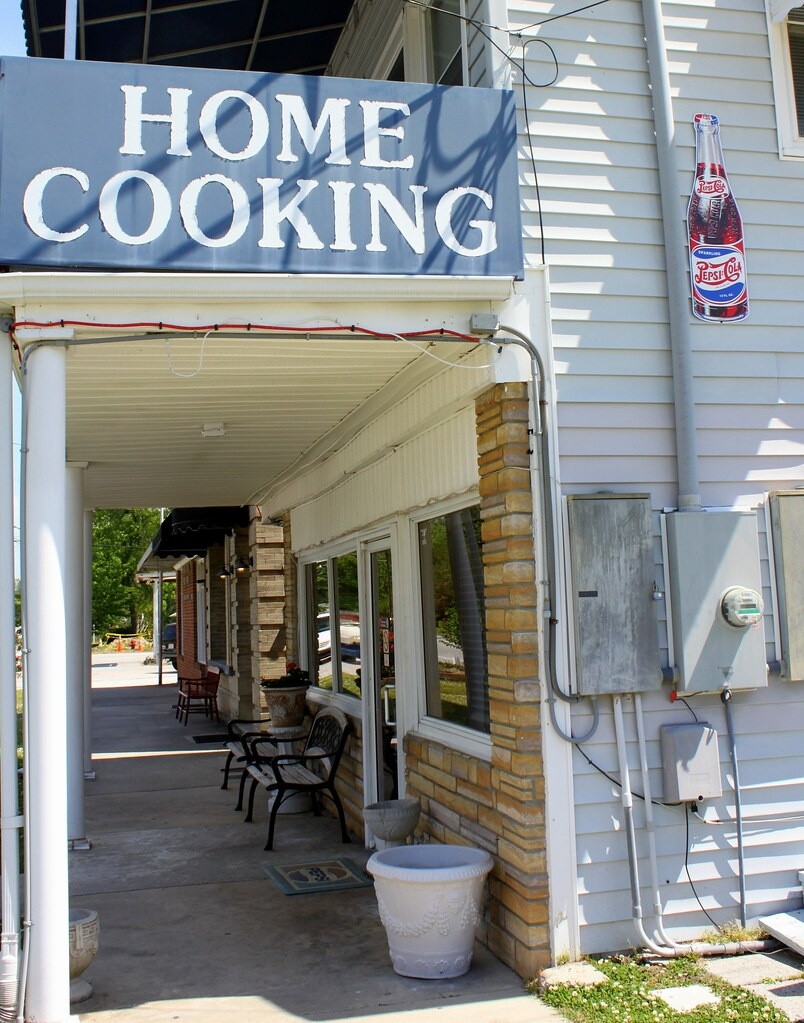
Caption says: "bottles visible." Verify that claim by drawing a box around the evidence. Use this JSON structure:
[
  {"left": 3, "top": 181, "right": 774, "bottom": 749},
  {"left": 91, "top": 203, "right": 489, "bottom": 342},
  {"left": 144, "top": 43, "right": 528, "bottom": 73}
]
[{"left": 687, "top": 114, "right": 750, "bottom": 323}]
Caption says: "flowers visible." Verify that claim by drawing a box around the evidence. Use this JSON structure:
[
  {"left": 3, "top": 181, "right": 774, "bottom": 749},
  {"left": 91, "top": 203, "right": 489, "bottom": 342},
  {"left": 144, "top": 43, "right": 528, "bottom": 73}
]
[{"left": 260, "top": 662, "right": 313, "bottom": 687}]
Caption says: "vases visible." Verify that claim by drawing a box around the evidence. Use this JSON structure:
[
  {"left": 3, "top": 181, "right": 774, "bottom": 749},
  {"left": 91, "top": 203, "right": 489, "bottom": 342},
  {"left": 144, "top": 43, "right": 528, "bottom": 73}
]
[
  {"left": 262, "top": 686, "right": 309, "bottom": 726},
  {"left": 366, "top": 842, "right": 495, "bottom": 979},
  {"left": 362, "top": 797, "right": 422, "bottom": 851},
  {"left": 68, "top": 910, "right": 100, "bottom": 1002}
]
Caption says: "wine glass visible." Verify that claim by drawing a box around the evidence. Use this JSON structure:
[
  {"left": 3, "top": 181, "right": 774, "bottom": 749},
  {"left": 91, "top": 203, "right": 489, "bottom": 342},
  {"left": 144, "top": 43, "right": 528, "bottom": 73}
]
[
  {"left": 363, "top": 799, "right": 421, "bottom": 849},
  {"left": 70, "top": 909, "right": 99, "bottom": 1002}
]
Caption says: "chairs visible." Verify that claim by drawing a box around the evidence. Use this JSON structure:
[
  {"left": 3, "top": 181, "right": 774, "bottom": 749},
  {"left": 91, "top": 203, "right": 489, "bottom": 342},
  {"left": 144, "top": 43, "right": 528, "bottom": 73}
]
[
  {"left": 221, "top": 718, "right": 275, "bottom": 810},
  {"left": 175, "top": 667, "right": 223, "bottom": 725},
  {"left": 235, "top": 708, "right": 352, "bottom": 852}
]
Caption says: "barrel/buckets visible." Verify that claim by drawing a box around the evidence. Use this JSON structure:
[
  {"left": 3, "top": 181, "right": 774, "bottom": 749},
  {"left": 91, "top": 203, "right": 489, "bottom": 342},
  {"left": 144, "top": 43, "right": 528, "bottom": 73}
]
[{"left": 366, "top": 845, "right": 495, "bottom": 979}]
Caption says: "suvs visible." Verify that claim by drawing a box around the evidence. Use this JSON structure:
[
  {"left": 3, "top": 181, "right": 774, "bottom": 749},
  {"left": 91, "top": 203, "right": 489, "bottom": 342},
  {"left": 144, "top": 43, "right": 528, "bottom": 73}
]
[
  {"left": 161, "top": 623, "right": 177, "bottom": 669},
  {"left": 316, "top": 612, "right": 331, "bottom": 659},
  {"left": 338, "top": 610, "right": 360, "bottom": 656}
]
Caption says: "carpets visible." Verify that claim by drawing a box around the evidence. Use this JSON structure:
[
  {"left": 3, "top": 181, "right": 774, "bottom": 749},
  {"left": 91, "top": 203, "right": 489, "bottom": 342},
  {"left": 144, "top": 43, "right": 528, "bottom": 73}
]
[
  {"left": 264, "top": 855, "right": 376, "bottom": 897},
  {"left": 192, "top": 732, "right": 239, "bottom": 744}
]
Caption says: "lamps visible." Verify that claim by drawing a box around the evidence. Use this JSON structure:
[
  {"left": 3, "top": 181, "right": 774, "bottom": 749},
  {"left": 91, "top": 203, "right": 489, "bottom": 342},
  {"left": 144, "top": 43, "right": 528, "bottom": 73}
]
[
  {"left": 236, "top": 556, "right": 254, "bottom": 573},
  {"left": 220, "top": 563, "right": 232, "bottom": 578}
]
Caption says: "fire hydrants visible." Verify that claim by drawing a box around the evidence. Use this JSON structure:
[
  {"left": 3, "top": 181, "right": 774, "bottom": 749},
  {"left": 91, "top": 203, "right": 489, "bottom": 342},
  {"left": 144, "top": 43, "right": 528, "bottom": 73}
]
[{"left": 130, "top": 639, "right": 136, "bottom": 649}]
